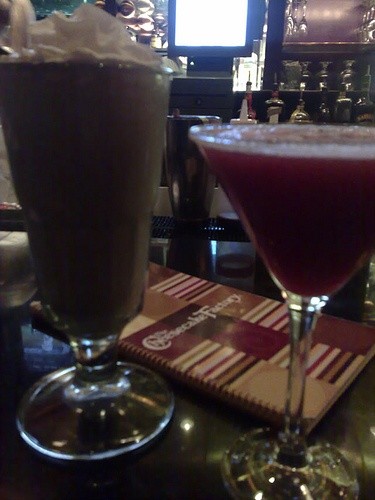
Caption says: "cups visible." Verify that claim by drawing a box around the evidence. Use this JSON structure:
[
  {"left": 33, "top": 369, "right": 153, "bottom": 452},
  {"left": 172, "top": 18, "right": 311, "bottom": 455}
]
[{"left": 166, "top": 115, "right": 222, "bottom": 227}]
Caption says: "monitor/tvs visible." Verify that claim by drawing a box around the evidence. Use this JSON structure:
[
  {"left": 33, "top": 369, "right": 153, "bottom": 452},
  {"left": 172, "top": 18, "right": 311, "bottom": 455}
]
[{"left": 167, "top": 0, "right": 255, "bottom": 73}]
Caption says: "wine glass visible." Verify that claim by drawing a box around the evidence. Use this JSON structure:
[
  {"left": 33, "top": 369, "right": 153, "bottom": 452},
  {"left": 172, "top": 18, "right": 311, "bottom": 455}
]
[
  {"left": 0, "top": 63, "right": 173, "bottom": 460},
  {"left": 190, "top": 124, "right": 375, "bottom": 500}
]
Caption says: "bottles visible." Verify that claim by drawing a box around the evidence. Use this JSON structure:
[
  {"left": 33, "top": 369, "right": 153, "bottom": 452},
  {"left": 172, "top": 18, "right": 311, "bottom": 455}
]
[
  {"left": 334, "top": 83, "right": 350, "bottom": 122},
  {"left": 150, "top": 30, "right": 157, "bottom": 48},
  {"left": 318, "top": 86, "right": 329, "bottom": 122},
  {"left": 265, "top": 82, "right": 283, "bottom": 121},
  {"left": 290, "top": 99, "right": 310, "bottom": 121}
]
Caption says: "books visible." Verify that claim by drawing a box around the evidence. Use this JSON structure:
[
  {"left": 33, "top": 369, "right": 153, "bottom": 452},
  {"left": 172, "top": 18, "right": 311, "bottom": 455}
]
[{"left": 27, "top": 256, "right": 375, "bottom": 435}]
